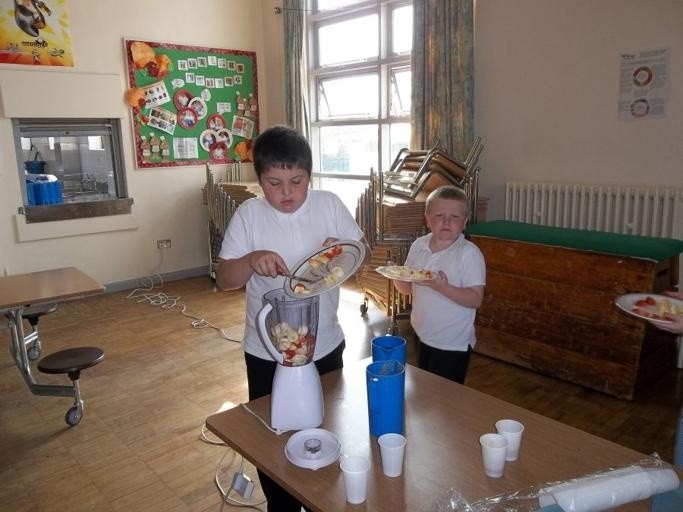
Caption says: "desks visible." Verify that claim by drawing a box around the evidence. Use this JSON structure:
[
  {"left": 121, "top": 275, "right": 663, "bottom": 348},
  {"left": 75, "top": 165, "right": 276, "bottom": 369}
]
[
  {"left": 206, "top": 351, "right": 683, "bottom": 512},
  {"left": 0, "top": 267, "right": 106, "bottom": 426}
]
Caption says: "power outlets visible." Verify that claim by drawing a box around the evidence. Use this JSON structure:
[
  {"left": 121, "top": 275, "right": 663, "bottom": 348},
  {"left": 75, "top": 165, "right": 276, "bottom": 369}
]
[{"left": 157, "top": 239, "right": 171, "bottom": 249}]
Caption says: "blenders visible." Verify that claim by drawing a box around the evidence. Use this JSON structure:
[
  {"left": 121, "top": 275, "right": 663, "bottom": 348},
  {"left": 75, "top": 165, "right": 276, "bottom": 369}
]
[{"left": 254, "top": 287, "right": 325, "bottom": 435}]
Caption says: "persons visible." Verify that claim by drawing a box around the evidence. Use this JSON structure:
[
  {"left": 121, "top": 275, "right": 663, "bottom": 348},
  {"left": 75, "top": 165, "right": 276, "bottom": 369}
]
[
  {"left": 214, "top": 126, "right": 373, "bottom": 512},
  {"left": 650, "top": 289, "right": 683, "bottom": 420},
  {"left": 387, "top": 187, "right": 487, "bottom": 385}
]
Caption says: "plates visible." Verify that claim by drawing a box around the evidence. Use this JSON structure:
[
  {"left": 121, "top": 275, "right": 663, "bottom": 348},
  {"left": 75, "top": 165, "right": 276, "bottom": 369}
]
[
  {"left": 374, "top": 265, "right": 440, "bottom": 283},
  {"left": 282, "top": 238, "right": 366, "bottom": 300},
  {"left": 612, "top": 292, "right": 682, "bottom": 324}
]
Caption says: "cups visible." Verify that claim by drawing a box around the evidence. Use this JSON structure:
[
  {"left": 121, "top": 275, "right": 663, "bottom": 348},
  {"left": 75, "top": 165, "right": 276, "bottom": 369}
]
[
  {"left": 477, "top": 419, "right": 525, "bottom": 480},
  {"left": 536, "top": 466, "right": 680, "bottom": 512},
  {"left": 339, "top": 454, "right": 369, "bottom": 505},
  {"left": 376, "top": 432, "right": 407, "bottom": 479}
]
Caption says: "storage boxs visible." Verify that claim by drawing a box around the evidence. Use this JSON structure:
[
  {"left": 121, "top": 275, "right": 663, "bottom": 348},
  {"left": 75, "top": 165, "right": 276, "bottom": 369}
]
[{"left": 26, "top": 180, "right": 63, "bottom": 207}]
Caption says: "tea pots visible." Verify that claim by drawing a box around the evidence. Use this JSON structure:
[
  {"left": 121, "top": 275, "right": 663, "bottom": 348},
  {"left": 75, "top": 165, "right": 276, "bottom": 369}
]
[{"left": 364, "top": 333, "right": 407, "bottom": 438}]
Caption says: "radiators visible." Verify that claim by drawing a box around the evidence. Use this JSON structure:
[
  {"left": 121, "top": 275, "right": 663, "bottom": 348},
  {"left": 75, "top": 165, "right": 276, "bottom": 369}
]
[{"left": 504, "top": 182, "right": 680, "bottom": 239}]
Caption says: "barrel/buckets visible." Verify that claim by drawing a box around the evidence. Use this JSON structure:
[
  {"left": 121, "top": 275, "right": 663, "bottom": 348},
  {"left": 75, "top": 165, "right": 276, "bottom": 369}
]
[{"left": 24, "top": 161, "right": 46, "bottom": 174}]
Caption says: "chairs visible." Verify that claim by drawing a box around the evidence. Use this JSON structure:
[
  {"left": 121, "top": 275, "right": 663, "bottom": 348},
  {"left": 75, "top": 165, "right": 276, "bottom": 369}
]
[{"left": 383, "top": 136, "right": 484, "bottom": 199}]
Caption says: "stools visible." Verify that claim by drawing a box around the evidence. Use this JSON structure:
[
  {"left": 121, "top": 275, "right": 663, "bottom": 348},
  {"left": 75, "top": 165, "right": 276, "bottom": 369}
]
[
  {"left": 3, "top": 302, "right": 58, "bottom": 361},
  {"left": 38, "top": 347, "right": 105, "bottom": 427}
]
